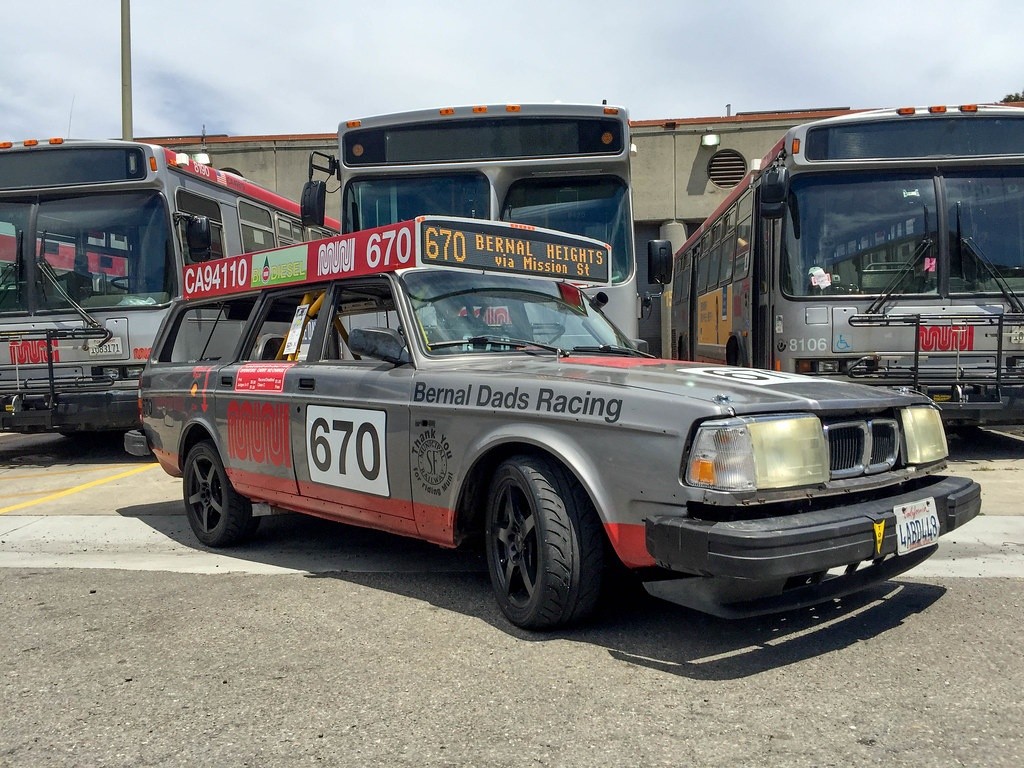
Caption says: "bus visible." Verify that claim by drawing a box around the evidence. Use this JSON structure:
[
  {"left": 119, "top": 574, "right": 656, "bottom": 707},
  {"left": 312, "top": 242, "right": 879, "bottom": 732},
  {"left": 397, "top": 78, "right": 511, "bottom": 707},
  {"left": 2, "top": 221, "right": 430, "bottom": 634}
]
[
  {"left": 0, "top": 212, "right": 130, "bottom": 295},
  {"left": 669, "top": 98, "right": 1024, "bottom": 429},
  {"left": 1, "top": 135, "right": 343, "bottom": 441},
  {"left": 299, "top": 102, "right": 676, "bottom": 352}
]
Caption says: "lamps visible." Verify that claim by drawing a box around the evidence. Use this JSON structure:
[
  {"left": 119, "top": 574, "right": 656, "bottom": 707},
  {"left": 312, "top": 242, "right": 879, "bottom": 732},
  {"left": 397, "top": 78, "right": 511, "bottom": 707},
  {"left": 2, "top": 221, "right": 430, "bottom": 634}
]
[
  {"left": 191, "top": 152, "right": 211, "bottom": 167},
  {"left": 700, "top": 133, "right": 720, "bottom": 146}
]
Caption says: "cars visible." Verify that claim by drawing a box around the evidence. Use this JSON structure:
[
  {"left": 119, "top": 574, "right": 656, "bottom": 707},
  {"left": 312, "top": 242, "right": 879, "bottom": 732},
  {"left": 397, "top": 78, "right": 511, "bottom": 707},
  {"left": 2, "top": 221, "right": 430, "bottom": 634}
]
[{"left": 121, "top": 214, "right": 984, "bottom": 638}]
